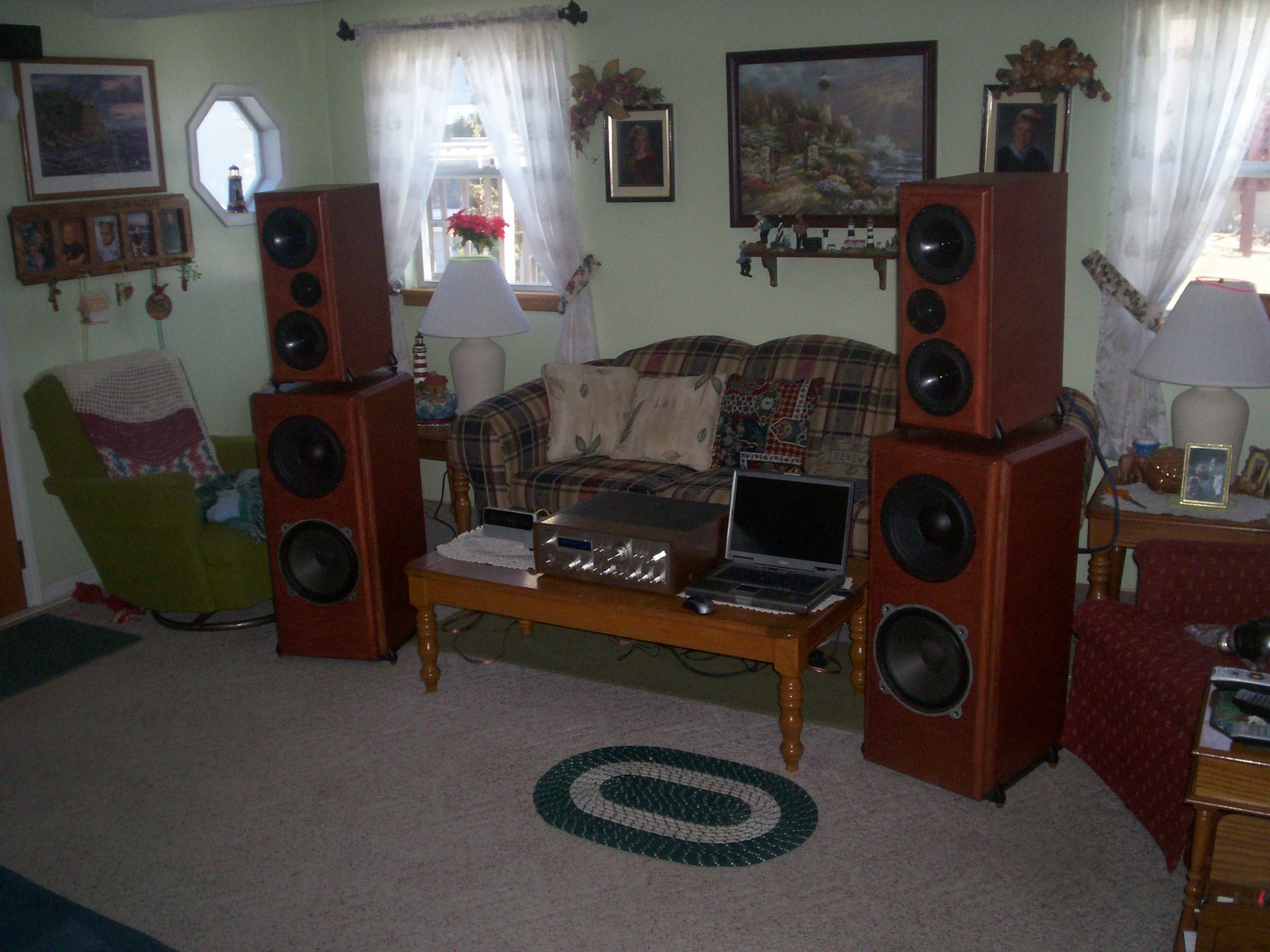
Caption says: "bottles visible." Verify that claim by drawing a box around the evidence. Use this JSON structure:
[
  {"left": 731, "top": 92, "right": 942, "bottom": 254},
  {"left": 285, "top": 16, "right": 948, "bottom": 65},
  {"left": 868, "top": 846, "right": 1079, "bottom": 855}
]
[
  {"left": 227, "top": 165, "right": 248, "bottom": 214},
  {"left": 413, "top": 331, "right": 428, "bottom": 383}
]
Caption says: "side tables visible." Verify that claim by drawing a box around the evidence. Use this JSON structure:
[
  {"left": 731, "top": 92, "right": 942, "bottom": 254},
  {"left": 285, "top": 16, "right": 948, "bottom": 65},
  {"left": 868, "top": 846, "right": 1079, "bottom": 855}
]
[{"left": 418, "top": 420, "right": 474, "bottom": 534}]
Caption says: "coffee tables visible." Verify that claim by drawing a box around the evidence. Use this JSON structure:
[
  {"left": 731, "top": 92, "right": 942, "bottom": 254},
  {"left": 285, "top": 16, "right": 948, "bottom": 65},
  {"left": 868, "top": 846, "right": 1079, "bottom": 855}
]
[{"left": 404, "top": 519, "right": 868, "bottom": 774}]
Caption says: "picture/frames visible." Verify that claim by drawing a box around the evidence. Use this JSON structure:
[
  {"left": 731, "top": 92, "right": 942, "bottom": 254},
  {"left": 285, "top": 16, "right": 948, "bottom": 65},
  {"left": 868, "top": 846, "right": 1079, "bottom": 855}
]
[
  {"left": 9, "top": 57, "right": 168, "bottom": 200},
  {"left": 1237, "top": 445, "right": 1270, "bottom": 497},
  {"left": 1178, "top": 442, "right": 1232, "bottom": 508},
  {"left": 725, "top": 40, "right": 937, "bottom": 228},
  {"left": 3, "top": 193, "right": 196, "bottom": 288},
  {"left": 605, "top": 101, "right": 674, "bottom": 204},
  {"left": 977, "top": 83, "right": 1075, "bottom": 173}
]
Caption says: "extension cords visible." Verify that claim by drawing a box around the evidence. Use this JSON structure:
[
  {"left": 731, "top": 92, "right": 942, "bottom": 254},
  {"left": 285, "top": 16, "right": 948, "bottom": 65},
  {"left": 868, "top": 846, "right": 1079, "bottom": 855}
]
[{"left": 481, "top": 507, "right": 536, "bottom": 533}]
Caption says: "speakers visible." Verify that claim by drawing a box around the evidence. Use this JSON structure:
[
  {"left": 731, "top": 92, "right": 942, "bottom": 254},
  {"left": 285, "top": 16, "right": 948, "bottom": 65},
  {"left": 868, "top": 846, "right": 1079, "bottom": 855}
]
[
  {"left": 252, "top": 181, "right": 400, "bottom": 391},
  {"left": 854, "top": 424, "right": 1093, "bottom": 809},
  {"left": 894, "top": 167, "right": 1068, "bottom": 439},
  {"left": 247, "top": 370, "right": 428, "bottom": 665}
]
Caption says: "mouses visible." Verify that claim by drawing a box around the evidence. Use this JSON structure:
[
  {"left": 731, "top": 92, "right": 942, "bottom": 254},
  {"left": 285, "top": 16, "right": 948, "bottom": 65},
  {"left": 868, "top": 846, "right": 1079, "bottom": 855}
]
[{"left": 683, "top": 594, "right": 716, "bottom": 615}]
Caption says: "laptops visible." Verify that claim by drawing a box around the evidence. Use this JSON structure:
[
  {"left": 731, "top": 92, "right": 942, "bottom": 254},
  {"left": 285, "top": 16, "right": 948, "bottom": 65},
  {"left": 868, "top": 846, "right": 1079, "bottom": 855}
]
[{"left": 683, "top": 469, "right": 856, "bottom": 616}]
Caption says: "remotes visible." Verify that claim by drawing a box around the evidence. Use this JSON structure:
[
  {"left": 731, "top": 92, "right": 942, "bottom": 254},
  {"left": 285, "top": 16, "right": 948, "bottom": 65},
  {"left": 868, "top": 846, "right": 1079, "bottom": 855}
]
[
  {"left": 1230, "top": 721, "right": 1270, "bottom": 744},
  {"left": 1232, "top": 690, "right": 1270, "bottom": 711},
  {"left": 1209, "top": 665, "right": 1270, "bottom": 693}
]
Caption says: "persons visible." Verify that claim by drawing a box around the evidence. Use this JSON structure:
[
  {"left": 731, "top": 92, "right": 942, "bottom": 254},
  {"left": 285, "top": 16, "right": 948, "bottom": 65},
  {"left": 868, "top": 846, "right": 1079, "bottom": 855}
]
[
  {"left": 751, "top": 211, "right": 772, "bottom": 243},
  {"left": 59, "top": 221, "right": 87, "bottom": 267},
  {"left": 22, "top": 224, "right": 53, "bottom": 270},
  {"left": 794, "top": 213, "right": 809, "bottom": 249},
  {"left": 97, "top": 221, "right": 120, "bottom": 261},
  {"left": 163, "top": 210, "right": 179, "bottom": 253},
  {"left": 736, "top": 241, "right": 752, "bottom": 278},
  {"left": 131, "top": 225, "right": 145, "bottom": 257},
  {"left": 618, "top": 124, "right": 663, "bottom": 186},
  {"left": 1186, "top": 457, "right": 1223, "bottom": 503}
]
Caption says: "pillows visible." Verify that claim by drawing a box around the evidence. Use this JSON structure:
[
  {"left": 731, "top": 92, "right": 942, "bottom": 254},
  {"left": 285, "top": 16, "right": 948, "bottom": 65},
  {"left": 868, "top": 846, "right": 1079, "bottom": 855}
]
[
  {"left": 537, "top": 358, "right": 639, "bottom": 462},
  {"left": 611, "top": 372, "right": 727, "bottom": 473},
  {"left": 705, "top": 374, "right": 827, "bottom": 478}
]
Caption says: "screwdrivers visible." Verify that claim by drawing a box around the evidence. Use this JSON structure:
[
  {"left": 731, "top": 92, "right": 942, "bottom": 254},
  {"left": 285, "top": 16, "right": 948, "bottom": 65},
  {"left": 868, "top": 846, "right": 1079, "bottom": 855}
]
[{"left": 1105, "top": 485, "right": 1147, "bottom": 509}]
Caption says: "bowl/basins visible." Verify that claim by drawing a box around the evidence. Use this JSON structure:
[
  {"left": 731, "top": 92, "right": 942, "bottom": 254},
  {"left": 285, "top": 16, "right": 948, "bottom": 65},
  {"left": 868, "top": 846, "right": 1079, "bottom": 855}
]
[{"left": 415, "top": 391, "right": 459, "bottom": 420}]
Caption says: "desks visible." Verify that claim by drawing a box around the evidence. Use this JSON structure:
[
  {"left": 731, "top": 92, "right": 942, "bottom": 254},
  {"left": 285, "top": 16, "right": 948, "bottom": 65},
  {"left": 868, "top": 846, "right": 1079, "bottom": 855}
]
[
  {"left": 1169, "top": 663, "right": 1270, "bottom": 952},
  {"left": 1084, "top": 462, "right": 1270, "bottom": 602}
]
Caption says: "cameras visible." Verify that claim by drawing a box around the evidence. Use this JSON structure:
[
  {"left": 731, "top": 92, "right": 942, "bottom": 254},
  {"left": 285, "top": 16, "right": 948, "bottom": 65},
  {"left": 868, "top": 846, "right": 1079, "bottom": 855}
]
[{"left": 1218, "top": 613, "right": 1270, "bottom": 660}]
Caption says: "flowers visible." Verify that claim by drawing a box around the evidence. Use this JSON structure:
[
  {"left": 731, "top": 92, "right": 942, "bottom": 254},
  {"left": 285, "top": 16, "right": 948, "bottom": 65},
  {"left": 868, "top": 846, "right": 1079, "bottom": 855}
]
[
  {"left": 447, "top": 208, "right": 510, "bottom": 257},
  {"left": 988, "top": 35, "right": 1114, "bottom": 107},
  {"left": 47, "top": 257, "right": 203, "bottom": 311},
  {"left": 566, "top": 62, "right": 665, "bottom": 162}
]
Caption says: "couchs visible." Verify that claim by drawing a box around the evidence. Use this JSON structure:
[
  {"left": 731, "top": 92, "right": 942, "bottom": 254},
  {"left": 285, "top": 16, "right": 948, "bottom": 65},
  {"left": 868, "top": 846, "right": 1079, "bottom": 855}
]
[
  {"left": 21, "top": 350, "right": 275, "bottom": 633},
  {"left": 1057, "top": 532, "right": 1270, "bottom": 873},
  {"left": 454, "top": 332, "right": 901, "bottom": 556}
]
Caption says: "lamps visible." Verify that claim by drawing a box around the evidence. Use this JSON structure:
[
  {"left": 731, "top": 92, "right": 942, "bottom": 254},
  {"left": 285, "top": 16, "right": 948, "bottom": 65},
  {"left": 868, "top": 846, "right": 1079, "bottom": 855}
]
[
  {"left": 1132, "top": 277, "right": 1270, "bottom": 485},
  {"left": 414, "top": 257, "right": 532, "bottom": 419}
]
